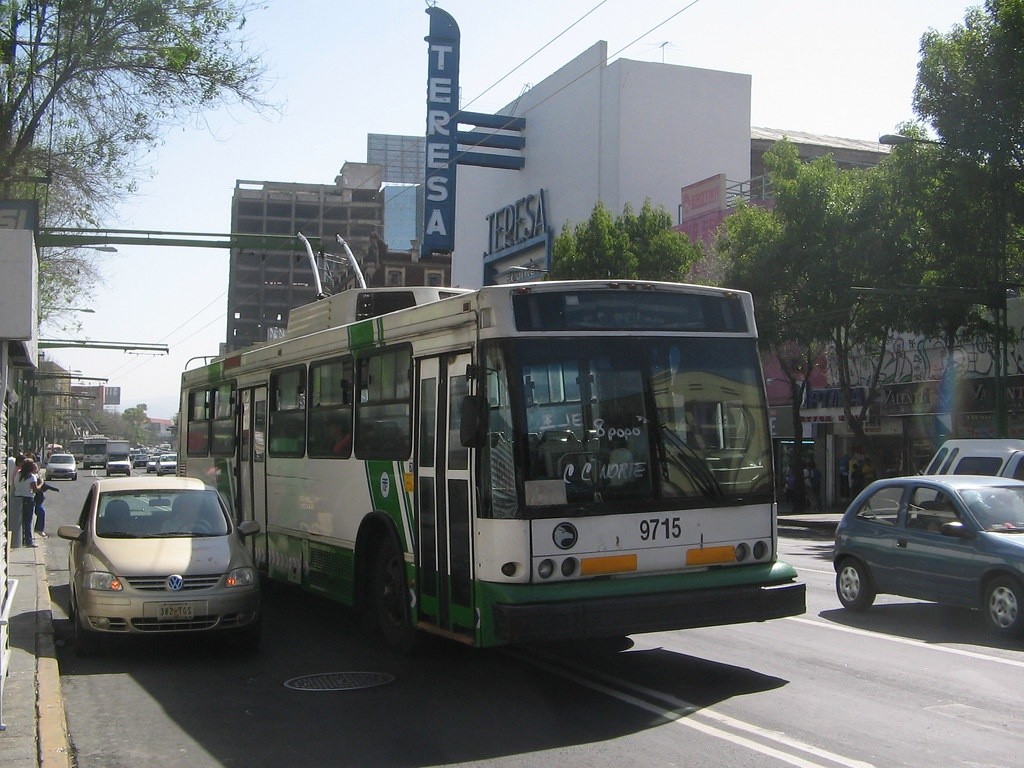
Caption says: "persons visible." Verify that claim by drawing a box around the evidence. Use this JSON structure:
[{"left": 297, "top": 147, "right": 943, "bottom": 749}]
[
  {"left": 5, "top": 446, "right": 59, "bottom": 548},
  {"left": 784, "top": 462, "right": 823, "bottom": 511},
  {"left": 160, "top": 493, "right": 212, "bottom": 532},
  {"left": 851, "top": 457, "right": 876, "bottom": 496},
  {"left": 606, "top": 417, "right": 657, "bottom": 489}
]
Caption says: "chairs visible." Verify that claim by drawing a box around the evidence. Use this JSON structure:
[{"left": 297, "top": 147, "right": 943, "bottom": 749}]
[
  {"left": 97, "top": 500, "right": 140, "bottom": 534},
  {"left": 376, "top": 420, "right": 599, "bottom": 493},
  {"left": 910, "top": 500, "right": 944, "bottom": 532},
  {"left": 159, "top": 496, "right": 183, "bottom": 533}
]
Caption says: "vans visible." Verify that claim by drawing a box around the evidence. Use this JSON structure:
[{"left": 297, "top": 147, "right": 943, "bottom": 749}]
[{"left": 912, "top": 437, "right": 1024, "bottom": 518}]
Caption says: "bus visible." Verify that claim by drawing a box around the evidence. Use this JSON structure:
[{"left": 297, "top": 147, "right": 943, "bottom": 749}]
[
  {"left": 169, "top": 230, "right": 810, "bottom": 658},
  {"left": 83, "top": 435, "right": 109, "bottom": 469}
]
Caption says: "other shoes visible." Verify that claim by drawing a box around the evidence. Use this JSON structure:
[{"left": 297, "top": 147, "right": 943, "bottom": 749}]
[
  {"left": 26, "top": 544, "right": 38, "bottom": 548},
  {"left": 34, "top": 528, "right": 46, "bottom": 537}
]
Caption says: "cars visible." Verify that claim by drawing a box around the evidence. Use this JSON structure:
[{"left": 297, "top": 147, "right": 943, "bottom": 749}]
[
  {"left": 44, "top": 452, "right": 79, "bottom": 481},
  {"left": 47, "top": 434, "right": 179, "bottom": 477},
  {"left": 832, "top": 473, "right": 1024, "bottom": 637},
  {"left": 57, "top": 475, "right": 266, "bottom": 649},
  {"left": 69, "top": 439, "right": 83, "bottom": 461}
]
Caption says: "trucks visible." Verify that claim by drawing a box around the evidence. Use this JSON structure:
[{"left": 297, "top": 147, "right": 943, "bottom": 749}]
[{"left": 103, "top": 440, "right": 132, "bottom": 477}]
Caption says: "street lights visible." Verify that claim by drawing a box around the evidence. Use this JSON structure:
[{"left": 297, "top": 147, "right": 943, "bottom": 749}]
[{"left": 877, "top": 133, "right": 1005, "bottom": 439}]
